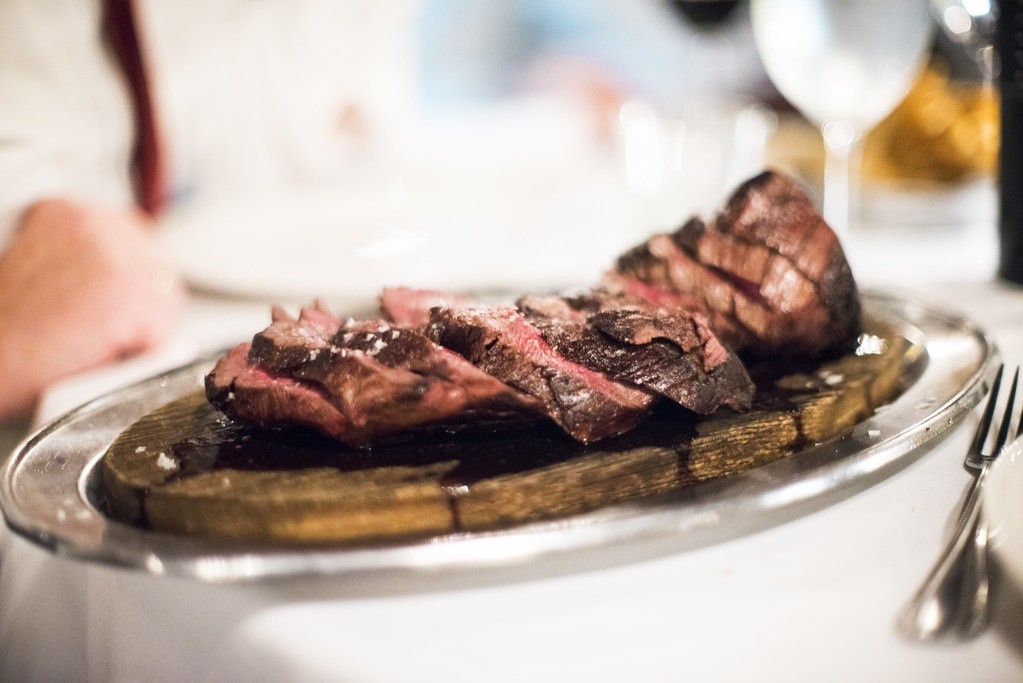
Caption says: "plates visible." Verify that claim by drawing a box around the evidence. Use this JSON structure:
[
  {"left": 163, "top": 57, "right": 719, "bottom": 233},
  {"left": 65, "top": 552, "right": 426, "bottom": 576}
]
[{"left": 2, "top": 295, "right": 1004, "bottom": 573}]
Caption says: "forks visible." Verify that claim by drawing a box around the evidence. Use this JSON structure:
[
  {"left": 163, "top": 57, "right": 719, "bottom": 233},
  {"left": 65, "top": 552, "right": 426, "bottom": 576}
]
[{"left": 897, "top": 364, "right": 1023, "bottom": 647}]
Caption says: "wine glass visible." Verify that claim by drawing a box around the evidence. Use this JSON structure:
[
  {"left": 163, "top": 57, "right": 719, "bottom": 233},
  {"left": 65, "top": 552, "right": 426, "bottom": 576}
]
[{"left": 750, "top": 1, "right": 932, "bottom": 249}]
